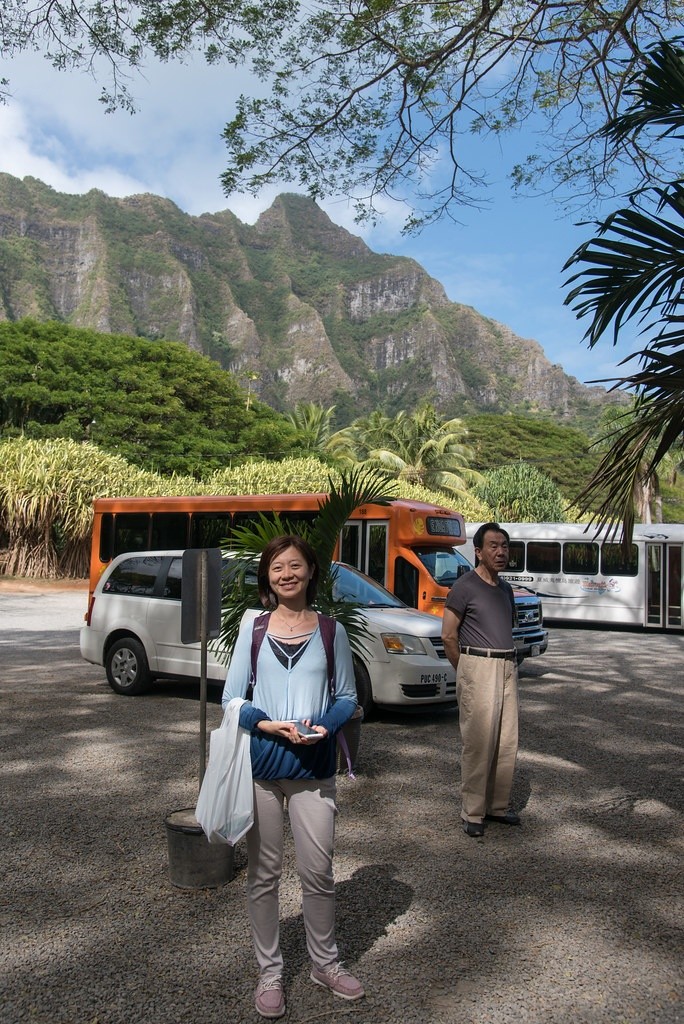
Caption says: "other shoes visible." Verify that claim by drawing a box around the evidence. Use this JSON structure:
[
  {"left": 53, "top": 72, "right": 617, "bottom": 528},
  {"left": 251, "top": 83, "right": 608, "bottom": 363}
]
[
  {"left": 462, "top": 819, "right": 484, "bottom": 836},
  {"left": 485, "top": 813, "right": 520, "bottom": 824}
]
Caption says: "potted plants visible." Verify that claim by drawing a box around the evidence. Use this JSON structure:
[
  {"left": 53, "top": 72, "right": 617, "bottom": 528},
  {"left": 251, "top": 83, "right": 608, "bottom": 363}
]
[{"left": 206, "top": 463, "right": 401, "bottom": 775}]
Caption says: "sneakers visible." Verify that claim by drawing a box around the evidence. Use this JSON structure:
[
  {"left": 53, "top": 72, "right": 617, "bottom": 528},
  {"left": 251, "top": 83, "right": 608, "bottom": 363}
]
[
  {"left": 310, "top": 961, "right": 364, "bottom": 1000},
  {"left": 255, "top": 975, "right": 285, "bottom": 1017}
]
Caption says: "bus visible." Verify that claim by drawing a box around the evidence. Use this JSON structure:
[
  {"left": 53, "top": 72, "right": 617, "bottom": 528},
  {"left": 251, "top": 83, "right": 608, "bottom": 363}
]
[
  {"left": 85, "top": 493, "right": 548, "bottom": 667},
  {"left": 449, "top": 523, "right": 684, "bottom": 629}
]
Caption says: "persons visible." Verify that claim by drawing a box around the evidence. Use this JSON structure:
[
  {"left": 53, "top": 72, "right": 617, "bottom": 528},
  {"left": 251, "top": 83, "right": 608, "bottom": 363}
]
[
  {"left": 222, "top": 535, "right": 365, "bottom": 1017},
  {"left": 441, "top": 522, "right": 521, "bottom": 837}
]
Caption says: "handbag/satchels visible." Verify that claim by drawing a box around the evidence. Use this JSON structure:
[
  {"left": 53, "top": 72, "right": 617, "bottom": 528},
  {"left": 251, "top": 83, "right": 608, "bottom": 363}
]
[{"left": 195, "top": 697, "right": 255, "bottom": 846}]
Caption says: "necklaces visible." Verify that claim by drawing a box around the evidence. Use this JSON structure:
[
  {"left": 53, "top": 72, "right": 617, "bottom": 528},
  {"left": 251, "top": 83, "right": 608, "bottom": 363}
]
[{"left": 274, "top": 610, "right": 315, "bottom": 631}]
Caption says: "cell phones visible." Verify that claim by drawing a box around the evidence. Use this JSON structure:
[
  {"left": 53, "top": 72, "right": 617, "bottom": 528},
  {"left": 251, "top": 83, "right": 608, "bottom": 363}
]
[{"left": 282, "top": 720, "right": 322, "bottom": 740}]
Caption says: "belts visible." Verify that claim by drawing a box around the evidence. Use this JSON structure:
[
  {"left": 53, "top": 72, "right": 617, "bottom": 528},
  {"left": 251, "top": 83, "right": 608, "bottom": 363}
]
[{"left": 461, "top": 648, "right": 516, "bottom": 660}]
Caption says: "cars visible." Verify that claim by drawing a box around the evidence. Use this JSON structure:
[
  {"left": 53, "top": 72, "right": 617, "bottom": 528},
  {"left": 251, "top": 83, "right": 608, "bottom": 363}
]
[{"left": 78, "top": 549, "right": 457, "bottom": 717}]
